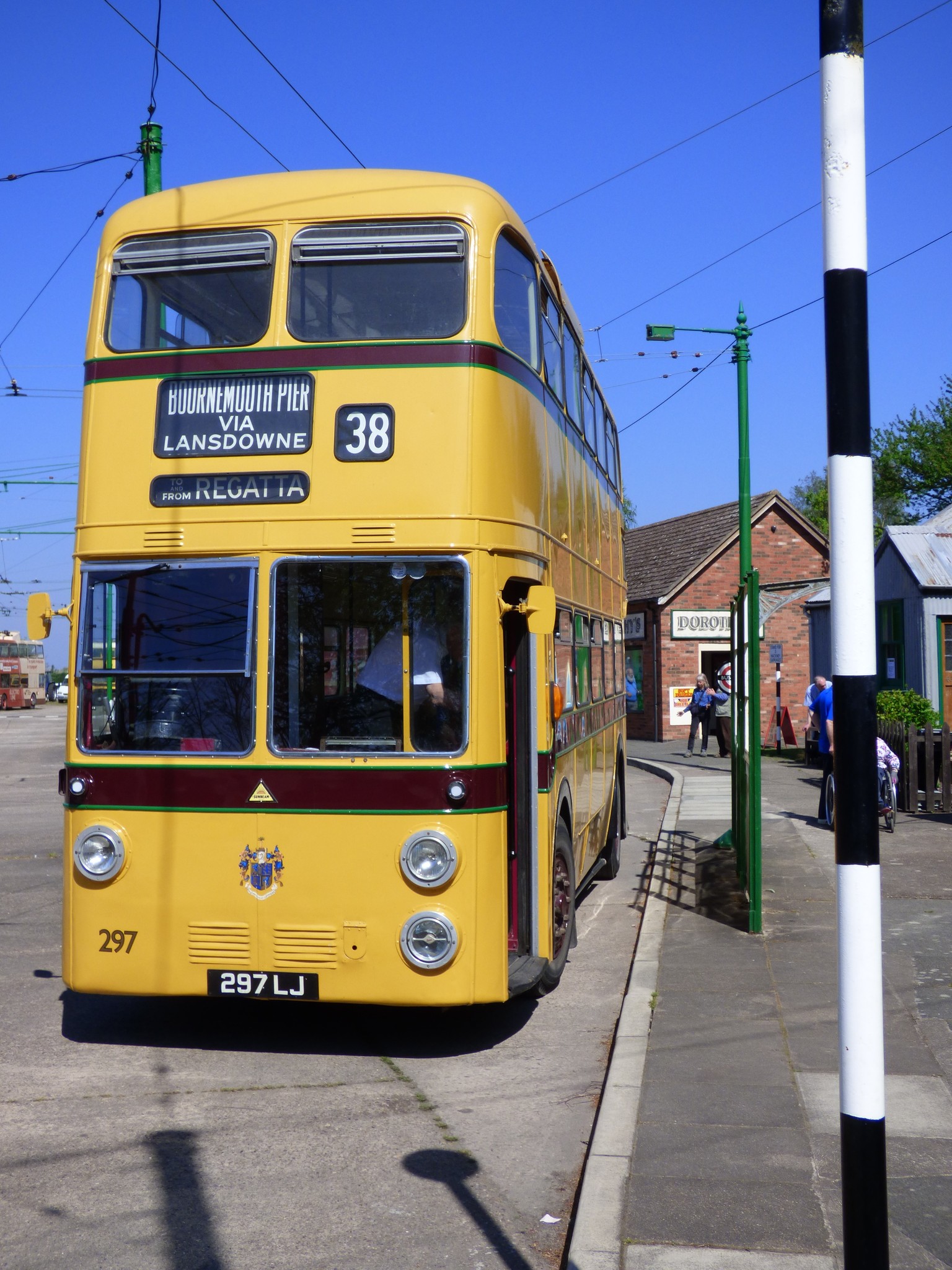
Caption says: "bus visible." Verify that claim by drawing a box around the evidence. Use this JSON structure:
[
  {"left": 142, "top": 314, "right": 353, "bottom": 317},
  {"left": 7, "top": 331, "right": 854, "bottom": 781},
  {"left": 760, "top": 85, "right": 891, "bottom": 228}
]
[
  {"left": 25, "top": 168, "right": 627, "bottom": 1011},
  {"left": 0, "top": 630, "right": 46, "bottom": 710},
  {"left": 93, "top": 640, "right": 117, "bottom": 700}
]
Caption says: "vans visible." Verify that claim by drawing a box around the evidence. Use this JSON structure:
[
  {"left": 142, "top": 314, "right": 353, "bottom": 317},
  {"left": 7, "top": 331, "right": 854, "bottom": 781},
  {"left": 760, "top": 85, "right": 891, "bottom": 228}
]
[{"left": 57, "top": 674, "right": 69, "bottom": 703}]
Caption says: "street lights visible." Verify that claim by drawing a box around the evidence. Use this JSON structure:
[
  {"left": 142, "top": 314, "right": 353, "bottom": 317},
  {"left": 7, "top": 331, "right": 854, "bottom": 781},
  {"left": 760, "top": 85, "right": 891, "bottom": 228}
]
[{"left": 641, "top": 299, "right": 764, "bottom": 585}]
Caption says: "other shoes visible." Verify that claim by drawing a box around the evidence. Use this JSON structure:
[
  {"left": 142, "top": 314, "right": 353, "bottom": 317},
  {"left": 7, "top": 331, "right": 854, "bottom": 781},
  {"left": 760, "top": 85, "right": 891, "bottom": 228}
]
[
  {"left": 818, "top": 818, "right": 829, "bottom": 825},
  {"left": 713, "top": 753, "right": 731, "bottom": 758},
  {"left": 879, "top": 804, "right": 890, "bottom": 814},
  {"left": 684, "top": 750, "right": 693, "bottom": 757},
  {"left": 700, "top": 749, "right": 707, "bottom": 757}
]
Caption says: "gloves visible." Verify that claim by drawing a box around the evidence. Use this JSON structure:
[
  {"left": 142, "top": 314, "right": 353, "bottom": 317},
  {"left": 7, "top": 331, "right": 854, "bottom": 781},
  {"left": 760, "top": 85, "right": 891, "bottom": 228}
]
[{"left": 890, "top": 770, "right": 899, "bottom": 784}]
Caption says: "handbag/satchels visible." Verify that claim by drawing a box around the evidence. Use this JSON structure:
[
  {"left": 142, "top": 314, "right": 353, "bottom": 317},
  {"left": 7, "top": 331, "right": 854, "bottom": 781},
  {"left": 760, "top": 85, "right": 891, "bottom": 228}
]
[{"left": 689, "top": 703, "right": 699, "bottom": 715}]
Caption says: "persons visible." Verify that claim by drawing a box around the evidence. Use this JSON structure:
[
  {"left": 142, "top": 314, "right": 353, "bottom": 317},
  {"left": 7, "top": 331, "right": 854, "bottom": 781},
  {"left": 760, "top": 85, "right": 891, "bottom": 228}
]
[
  {"left": 803, "top": 675, "right": 832, "bottom": 761},
  {"left": 625, "top": 668, "right": 642, "bottom": 713},
  {"left": 706, "top": 669, "right": 732, "bottom": 758},
  {"left": 676, "top": 673, "right": 714, "bottom": 757},
  {"left": 877, "top": 737, "right": 899, "bottom": 815},
  {"left": 340, "top": 611, "right": 463, "bottom": 752},
  {"left": 808, "top": 684, "right": 834, "bottom": 824}
]
